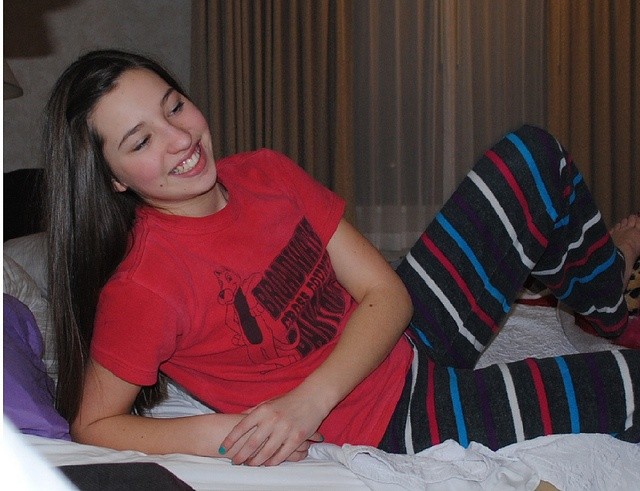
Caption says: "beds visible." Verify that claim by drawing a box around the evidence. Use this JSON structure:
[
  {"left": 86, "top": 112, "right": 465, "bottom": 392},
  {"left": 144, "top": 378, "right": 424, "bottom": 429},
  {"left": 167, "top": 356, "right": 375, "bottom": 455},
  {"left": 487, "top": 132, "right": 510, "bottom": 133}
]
[{"left": 4, "top": 166, "right": 640, "bottom": 491}]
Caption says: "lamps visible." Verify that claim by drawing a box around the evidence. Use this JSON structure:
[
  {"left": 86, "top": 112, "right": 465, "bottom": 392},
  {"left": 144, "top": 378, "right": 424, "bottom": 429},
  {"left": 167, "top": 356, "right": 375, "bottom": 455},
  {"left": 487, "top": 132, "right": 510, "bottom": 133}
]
[{"left": 4, "top": 58, "right": 24, "bottom": 101}]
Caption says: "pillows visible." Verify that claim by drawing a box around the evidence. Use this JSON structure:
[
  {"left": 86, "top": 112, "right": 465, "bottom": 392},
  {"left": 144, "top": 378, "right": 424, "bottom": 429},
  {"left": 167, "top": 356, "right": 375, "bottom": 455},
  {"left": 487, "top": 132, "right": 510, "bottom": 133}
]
[
  {"left": 3, "top": 232, "right": 51, "bottom": 297},
  {"left": 4, "top": 293, "right": 71, "bottom": 437},
  {"left": 2, "top": 251, "right": 59, "bottom": 363}
]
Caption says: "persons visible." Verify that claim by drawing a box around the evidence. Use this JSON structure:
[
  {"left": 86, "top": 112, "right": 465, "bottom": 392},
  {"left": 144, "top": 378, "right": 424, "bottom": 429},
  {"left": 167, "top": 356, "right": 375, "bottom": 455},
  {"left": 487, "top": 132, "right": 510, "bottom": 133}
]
[{"left": 37, "top": 49, "right": 640, "bottom": 467}]
